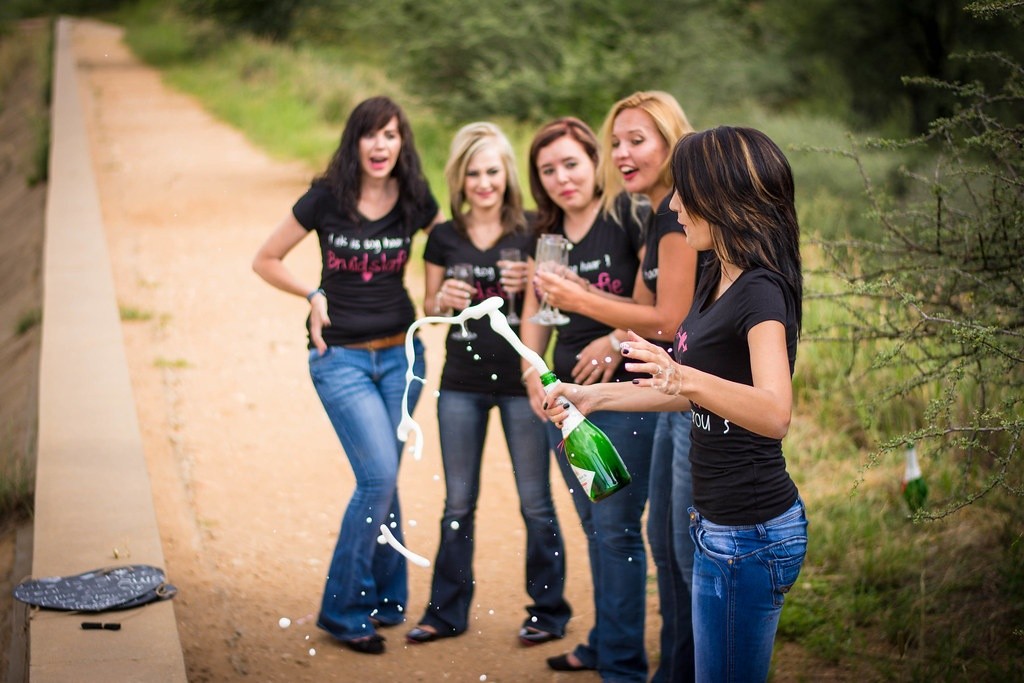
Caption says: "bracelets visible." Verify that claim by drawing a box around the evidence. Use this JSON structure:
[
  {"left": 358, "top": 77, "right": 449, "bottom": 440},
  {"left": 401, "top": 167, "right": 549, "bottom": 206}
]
[
  {"left": 433, "top": 292, "right": 444, "bottom": 315},
  {"left": 583, "top": 278, "right": 591, "bottom": 292},
  {"left": 609, "top": 334, "right": 621, "bottom": 353},
  {"left": 521, "top": 365, "right": 536, "bottom": 384}
]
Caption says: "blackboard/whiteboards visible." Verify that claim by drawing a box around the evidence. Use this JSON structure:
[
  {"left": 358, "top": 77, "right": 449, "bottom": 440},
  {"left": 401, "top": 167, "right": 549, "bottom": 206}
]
[{"left": 14, "top": 563, "right": 165, "bottom": 611}]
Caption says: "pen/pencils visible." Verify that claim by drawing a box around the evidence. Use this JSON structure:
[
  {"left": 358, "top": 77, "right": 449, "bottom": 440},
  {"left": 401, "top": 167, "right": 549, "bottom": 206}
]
[{"left": 82, "top": 621, "right": 121, "bottom": 630}]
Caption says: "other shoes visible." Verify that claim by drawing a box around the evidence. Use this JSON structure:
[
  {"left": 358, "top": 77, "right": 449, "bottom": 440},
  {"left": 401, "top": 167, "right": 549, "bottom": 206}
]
[
  {"left": 366, "top": 616, "right": 386, "bottom": 628},
  {"left": 346, "top": 634, "right": 385, "bottom": 654},
  {"left": 547, "top": 653, "right": 591, "bottom": 671}
]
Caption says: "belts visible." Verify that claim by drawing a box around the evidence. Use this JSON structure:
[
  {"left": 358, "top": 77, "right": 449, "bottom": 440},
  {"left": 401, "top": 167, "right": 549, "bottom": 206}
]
[{"left": 343, "top": 331, "right": 416, "bottom": 349}]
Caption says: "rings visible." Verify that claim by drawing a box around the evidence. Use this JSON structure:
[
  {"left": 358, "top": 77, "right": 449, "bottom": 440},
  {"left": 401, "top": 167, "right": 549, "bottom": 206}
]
[
  {"left": 544, "top": 291, "right": 548, "bottom": 301},
  {"left": 458, "top": 281, "right": 464, "bottom": 289},
  {"left": 650, "top": 365, "right": 662, "bottom": 376},
  {"left": 522, "top": 277, "right": 527, "bottom": 285}
]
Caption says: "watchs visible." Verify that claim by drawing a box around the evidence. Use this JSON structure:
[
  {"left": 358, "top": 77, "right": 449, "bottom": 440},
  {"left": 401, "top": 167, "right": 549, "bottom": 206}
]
[{"left": 307, "top": 289, "right": 326, "bottom": 304}]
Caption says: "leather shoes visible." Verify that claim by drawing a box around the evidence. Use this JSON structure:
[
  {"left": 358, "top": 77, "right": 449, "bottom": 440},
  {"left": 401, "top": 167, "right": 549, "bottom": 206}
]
[
  {"left": 406, "top": 625, "right": 445, "bottom": 643},
  {"left": 519, "top": 626, "right": 563, "bottom": 644}
]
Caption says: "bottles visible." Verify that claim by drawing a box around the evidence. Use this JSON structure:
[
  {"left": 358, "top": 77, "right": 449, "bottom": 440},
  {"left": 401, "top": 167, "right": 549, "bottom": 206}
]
[
  {"left": 541, "top": 370, "right": 632, "bottom": 504},
  {"left": 900, "top": 443, "right": 931, "bottom": 512}
]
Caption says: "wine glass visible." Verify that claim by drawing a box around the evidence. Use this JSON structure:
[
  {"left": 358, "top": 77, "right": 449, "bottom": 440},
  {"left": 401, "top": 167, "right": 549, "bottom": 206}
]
[
  {"left": 448, "top": 263, "right": 479, "bottom": 338},
  {"left": 526, "top": 232, "right": 571, "bottom": 326},
  {"left": 502, "top": 248, "right": 525, "bottom": 325}
]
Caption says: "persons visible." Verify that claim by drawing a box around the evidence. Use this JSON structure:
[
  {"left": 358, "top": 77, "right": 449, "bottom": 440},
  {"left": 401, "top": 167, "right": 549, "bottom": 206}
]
[
  {"left": 253, "top": 97, "right": 446, "bottom": 655},
  {"left": 519, "top": 90, "right": 710, "bottom": 683},
  {"left": 538, "top": 125, "right": 809, "bottom": 683},
  {"left": 404, "top": 122, "right": 573, "bottom": 646}
]
